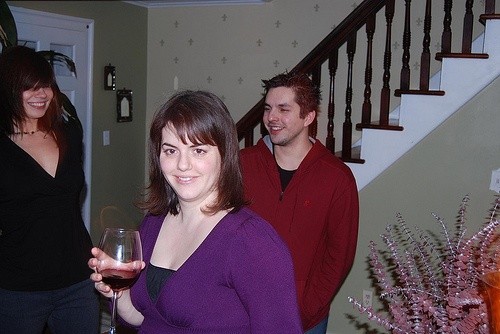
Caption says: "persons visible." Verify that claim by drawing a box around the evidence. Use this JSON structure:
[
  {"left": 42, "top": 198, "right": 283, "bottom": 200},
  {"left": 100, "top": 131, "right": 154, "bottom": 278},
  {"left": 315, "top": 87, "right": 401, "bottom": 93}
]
[
  {"left": 239, "top": 74, "right": 359, "bottom": 334},
  {"left": 88, "top": 90, "right": 303, "bottom": 334},
  {"left": 0, "top": 46, "right": 102, "bottom": 334}
]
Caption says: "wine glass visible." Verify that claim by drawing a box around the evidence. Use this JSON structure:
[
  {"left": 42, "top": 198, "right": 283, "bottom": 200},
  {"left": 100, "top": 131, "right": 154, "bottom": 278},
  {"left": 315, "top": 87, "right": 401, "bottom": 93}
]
[{"left": 95, "top": 228, "right": 142, "bottom": 334}]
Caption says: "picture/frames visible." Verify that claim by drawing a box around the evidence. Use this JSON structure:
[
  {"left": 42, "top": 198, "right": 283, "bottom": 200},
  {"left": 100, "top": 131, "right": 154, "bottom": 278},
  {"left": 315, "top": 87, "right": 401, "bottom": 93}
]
[
  {"left": 116, "top": 89, "right": 134, "bottom": 122},
  {"left": 105, "top": 65, "right": 117, "bottom": 92}
]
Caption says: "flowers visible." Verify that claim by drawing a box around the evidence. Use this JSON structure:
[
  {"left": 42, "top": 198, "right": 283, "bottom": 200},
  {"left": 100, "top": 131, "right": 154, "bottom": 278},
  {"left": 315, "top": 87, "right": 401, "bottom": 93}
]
[{"left": 348, "top": 191, "right": 500, "bottom": 334}]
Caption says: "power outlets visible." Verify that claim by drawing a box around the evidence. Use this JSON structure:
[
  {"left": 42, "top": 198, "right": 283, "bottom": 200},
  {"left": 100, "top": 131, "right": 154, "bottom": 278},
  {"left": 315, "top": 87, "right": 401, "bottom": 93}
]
[{"left": 362, "top": 290, "right": 373, "bottom": 308}]
